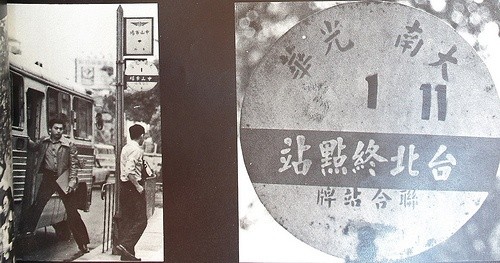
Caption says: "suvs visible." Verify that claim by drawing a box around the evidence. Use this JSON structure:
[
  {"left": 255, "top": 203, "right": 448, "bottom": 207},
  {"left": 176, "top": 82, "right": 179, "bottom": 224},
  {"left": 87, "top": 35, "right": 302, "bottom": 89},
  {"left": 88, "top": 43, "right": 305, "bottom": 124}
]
[{"left": 142, "top": 152, "right": 163, "bottom": 186}]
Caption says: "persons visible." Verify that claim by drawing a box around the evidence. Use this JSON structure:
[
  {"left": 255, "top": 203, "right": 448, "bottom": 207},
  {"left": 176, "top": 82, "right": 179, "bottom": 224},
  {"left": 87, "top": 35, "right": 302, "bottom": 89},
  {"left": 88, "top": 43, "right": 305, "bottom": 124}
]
[
  {"left": 111, "top": 124, "right": 153, "bottom": 260},
  {"left": 23, "top": 118, "right": 90, "bottom": 254}
]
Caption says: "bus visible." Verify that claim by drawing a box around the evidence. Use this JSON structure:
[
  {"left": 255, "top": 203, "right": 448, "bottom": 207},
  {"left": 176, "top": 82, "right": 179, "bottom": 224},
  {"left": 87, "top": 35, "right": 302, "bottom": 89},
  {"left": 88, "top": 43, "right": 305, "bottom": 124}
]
[
  {"left": 6, "top": 53, "right": 105, "bottom": 240},
  {"left": 93, "top": 142, "right": 117, "bottom": 186}
]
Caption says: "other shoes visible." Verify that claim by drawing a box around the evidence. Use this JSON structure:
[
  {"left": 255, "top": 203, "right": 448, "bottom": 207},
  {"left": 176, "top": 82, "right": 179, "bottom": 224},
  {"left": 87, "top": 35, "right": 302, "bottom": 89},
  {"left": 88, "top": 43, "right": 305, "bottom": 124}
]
[
  {"left": 116, "top": 243, "right": 140, "bottom": 259},
  {"left": 120, "top": 253, "right": 133, "bottom": 260}
]
